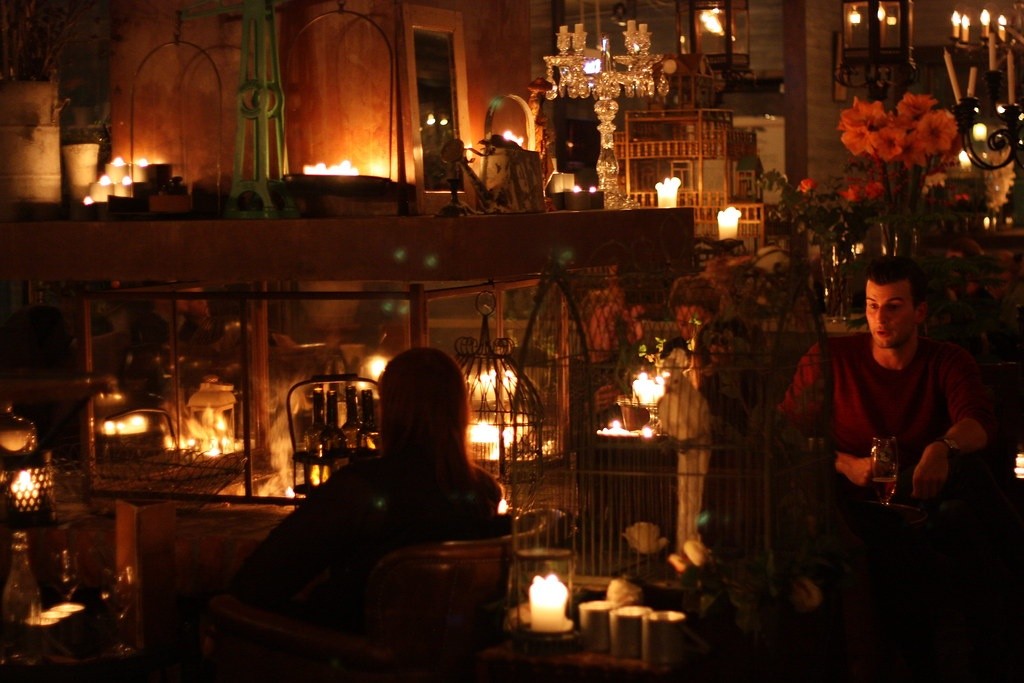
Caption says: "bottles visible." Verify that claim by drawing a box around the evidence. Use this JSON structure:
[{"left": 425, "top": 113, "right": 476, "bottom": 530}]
[
  {"left": 302, "top": 385, "right": 380, "bottom": 488},
  {"left": 3, "top": 533, "right": 42, "bottom": 664}
]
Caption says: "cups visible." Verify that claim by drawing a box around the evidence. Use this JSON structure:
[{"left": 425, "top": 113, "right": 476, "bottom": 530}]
[
  {"left": 642, "top": 610, "right": 688, "bottom": 663},
  {"left": 578, "top": 600, "right": 623, "bottom": 654},
  {"left": 61, "top": 143, "right": 100, "bottom": 202},
  {"left": 607, "top": 605, "right": 653, "bottom": 658}
]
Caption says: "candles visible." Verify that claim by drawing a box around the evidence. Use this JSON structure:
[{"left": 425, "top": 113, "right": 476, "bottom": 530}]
[
  {"left": 989, "top": 31, "right": 997, "bottom": 70},
  {"left": 575, "top": 23, "right": 584, "bottom": 35},
  {"left": 529, "top": 578, "right": 568, "bottom": 628},
  {"left": 1007, "top": 50, "right": 1015, "bottom": 106},
  {"left": 626, "top": 20, "right": 636, "bottom": 32},
  {"left": 941, "top": 47, "right": 961, "bottom": 103},
  {"left": 639, "top": 23, "right": 648, "bottom": 32},
  {"left": 559, "top": 24, "right": 568, "bottom": 34},
  {"left": 968, "top": 66, "right": 978, "bottom": 96}
]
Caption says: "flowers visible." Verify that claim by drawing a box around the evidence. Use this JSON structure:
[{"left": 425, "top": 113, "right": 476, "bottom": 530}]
[
  {"left": 622, "top": 522, "right": 717, "bottom": 600},
  {"left": 735, "top": 153, "right": 976, "bottom": 317},
  {"left": 837, "top": 93, "right": 959, "bottom": 257}
]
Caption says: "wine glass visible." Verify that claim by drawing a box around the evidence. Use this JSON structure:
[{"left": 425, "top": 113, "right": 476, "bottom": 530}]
[{"left": 871, "top": 437, "right": 900, "bottom": 507}]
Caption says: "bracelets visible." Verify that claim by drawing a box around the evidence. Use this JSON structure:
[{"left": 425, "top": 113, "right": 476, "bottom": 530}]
[{"left": 938, "top": 437, "right": 960, "bottom": 454}]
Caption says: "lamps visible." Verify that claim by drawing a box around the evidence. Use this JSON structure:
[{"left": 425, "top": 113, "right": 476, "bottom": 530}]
[
  {"left": 0, "top": 403, "right": 56, "bottom": 531},
  {"left": 832, "top": 1, "right": 920, "bottom": 100},
  {"left": 947, "top": 10, "right": 1024, "bottom": 171},
  {"left": 675, "top": 0, "right": 752, "bottom": 78}
]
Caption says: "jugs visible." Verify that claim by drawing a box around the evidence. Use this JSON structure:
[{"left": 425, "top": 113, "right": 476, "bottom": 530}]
[{"left": 443, "top": 95, "right": 548, "bottom": 215}]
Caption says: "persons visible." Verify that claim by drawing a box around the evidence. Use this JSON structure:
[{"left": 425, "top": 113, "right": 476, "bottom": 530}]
[
  {"left": 1, "top": 302, "right": 100, "bottom": 657},
  {"left": 696, "top": 316, "right": 804, "bottom": 444},
  {"left": 229, "top": 348, "right": 500, "bottom": 683},
  {"left": 785, "top": 256, "right": 998, "bottom": 499}
]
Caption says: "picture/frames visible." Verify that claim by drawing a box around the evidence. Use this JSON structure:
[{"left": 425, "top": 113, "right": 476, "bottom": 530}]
[{"left": 399, "top": 3, "right": 475, "bottom": 216}]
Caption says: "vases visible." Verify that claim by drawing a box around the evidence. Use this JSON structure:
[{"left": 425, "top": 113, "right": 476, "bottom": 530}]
[
  {"left": 881, "top": 218, "right": 916, "bottom": 259},
  {"left": 821, "top": 249, "right": 851, "bottom": 321}
]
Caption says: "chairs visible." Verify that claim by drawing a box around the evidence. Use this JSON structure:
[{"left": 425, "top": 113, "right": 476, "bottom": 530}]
[{"left": 207, "top": 508, "right": 572, "bottom": 683}]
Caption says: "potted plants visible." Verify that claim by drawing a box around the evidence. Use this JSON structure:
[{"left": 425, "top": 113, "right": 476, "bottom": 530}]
[
  {"left": 921, "top": 254, "right": 1010, "bottom": 353},
  {"left": 0, "top": 0, "right": 127, "bottom": 217},
  {"left": 616, "top": 315, "right": 702, "bottom": 431}
]
